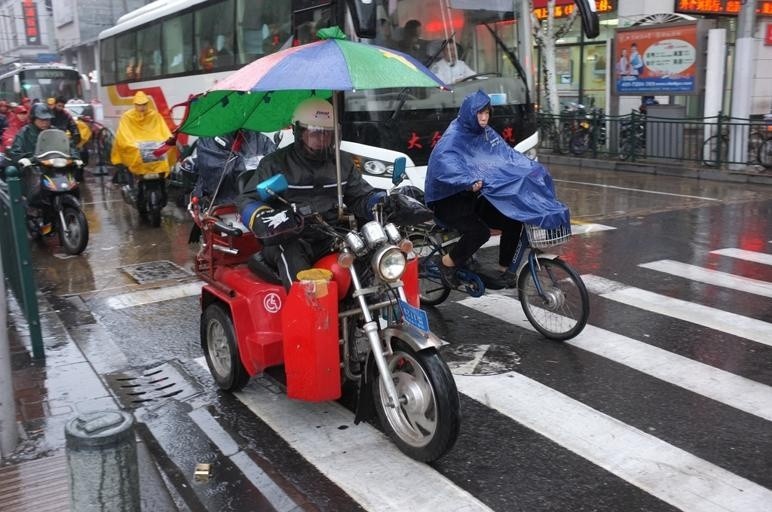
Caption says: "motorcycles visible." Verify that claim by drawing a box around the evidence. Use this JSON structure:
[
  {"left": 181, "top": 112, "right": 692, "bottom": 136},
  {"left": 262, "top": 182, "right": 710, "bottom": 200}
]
[
  {"left": 110, "top": 138, "right": 174, "bottom": 231},
  {"left": 538, "top": 101, "right": 644, "bottom": 158},
  {"left": 15, "top": 133, "right": 91, "bottom": 261}
]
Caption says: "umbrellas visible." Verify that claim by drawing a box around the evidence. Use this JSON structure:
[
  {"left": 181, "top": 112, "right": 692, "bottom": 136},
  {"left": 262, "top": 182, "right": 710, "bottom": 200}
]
[{"left": 170, "top": 26, "right": 453, "bottom": 216}]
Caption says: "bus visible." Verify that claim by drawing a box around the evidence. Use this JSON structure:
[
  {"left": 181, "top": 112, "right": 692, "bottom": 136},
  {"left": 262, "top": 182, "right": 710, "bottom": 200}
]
[
  {"left": 0, "top": 63, "right": 91, "bottom": 108},
  {"left": 93, "top": 0, "right": 603, "bottom": 212}
]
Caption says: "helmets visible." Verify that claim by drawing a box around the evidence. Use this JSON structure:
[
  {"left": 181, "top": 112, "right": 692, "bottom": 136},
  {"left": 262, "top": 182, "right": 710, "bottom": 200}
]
[{"left": 28, "top": 103, "right": 56, "bottom": 119}]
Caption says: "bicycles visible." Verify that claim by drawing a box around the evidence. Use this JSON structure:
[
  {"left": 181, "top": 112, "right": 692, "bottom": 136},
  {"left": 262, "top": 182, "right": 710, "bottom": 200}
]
[
  {"left": 402, "top": 196, "right": 589, "bottom": 344},
  {"left": 698, "top": 112, "right": 765, "bottom": 174},
  {"left": 754, "top": 109, "right": 772, "bottom": 169}
]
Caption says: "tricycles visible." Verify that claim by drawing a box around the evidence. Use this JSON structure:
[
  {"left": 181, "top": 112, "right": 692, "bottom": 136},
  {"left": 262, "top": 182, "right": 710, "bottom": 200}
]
[{"left": 191, "top": 185, "right": 461, "bottom": 466}]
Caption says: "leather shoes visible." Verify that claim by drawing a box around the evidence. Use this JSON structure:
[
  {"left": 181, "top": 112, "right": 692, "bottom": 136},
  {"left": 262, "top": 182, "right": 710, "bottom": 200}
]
[
  {"left": 32, "top": 206, "right": 38, "bottom": 217},
  {"left": 435, "top": 255, "right": 458, "bottom": 289}
]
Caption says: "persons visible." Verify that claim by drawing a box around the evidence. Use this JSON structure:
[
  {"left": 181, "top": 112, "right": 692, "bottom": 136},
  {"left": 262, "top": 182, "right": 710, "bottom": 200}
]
[
  {"left": 127, "top": 57, "right": 142, "bottom": 80},
  {"left": 0, "top": 96, "right": 80, "bottom": 234},
  {"left": 238, "top": 97, "right": 384, "bottom": 294},
  {"left": 200, "top": 42, "right": 225, "bottom": 72},
  {"left": 264, "top": 24, "right": 289, "bottom": 56},
  {"left": 193, "top": 133, "right": 274, "bottom": 196},
  {"left": 116, "top": 91, "right": 175, "bottom": 156},
  {"left": 427, "top": 91, "right": 541, "bottom": 289},
  {"left": 617, "top": 45, "right": 644, "bottom": 76},
  {"left": 400, "top": 20, "right": 431, "bottom": 64},
  {"left": 425, "top": 39, "right": 476, "bottom": 94}
]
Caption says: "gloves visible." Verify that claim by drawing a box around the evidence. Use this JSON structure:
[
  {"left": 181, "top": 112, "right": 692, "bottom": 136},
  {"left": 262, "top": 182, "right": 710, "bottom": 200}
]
[
  {"left": 17, "top": 158, "right": 31, "bottom": 166},
  {"left": 74, "top": 160, "right": 83, "bottom": 165}
]
